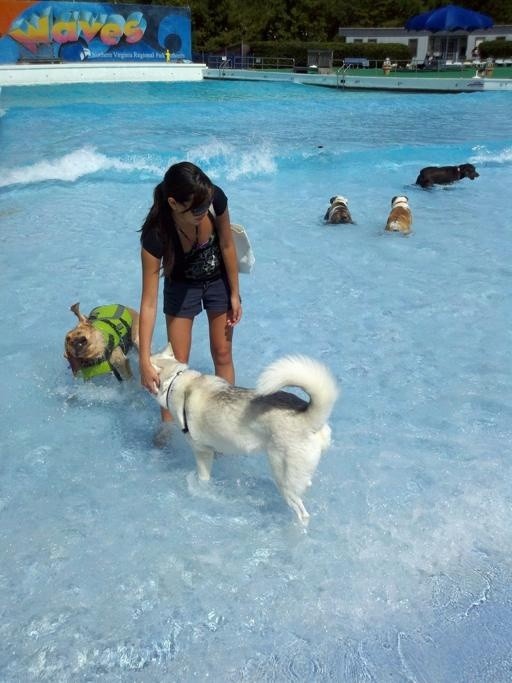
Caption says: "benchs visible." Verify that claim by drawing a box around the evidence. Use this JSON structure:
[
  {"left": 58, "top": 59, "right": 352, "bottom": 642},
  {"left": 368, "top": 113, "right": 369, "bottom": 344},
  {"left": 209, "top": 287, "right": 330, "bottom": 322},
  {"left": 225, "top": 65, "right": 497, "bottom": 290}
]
[
  {"left": 293, "top": 65, "right": 318, "bottom": 74},
  {"left": 343, "top": 57, "right": 368, "bottom": 70}
]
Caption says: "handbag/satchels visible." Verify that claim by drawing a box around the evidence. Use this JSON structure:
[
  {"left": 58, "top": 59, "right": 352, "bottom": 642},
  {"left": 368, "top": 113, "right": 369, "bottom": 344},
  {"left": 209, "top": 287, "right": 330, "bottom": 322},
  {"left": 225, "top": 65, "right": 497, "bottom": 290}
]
[{"left": 207, "top": 201, "right": 254, "bottom": 273}]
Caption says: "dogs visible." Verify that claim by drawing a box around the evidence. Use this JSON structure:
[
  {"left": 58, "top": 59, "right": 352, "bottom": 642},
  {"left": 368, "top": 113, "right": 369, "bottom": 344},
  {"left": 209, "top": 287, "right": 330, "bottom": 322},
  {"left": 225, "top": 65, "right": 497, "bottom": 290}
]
[
  {"left": 64, "top": 303, "right": 140, "bottom": 384},
  {"left": 141, "top": 342, "right": 338, "bottom": 534},
  {"left": 416, "top": 164, "right": 479, "bottom": 193},
  {"left": 385, "top": 196, "right": 412, "bottom": 234},
  {"left": 325, "top": 196, "right": 353, "bottom": 223}
]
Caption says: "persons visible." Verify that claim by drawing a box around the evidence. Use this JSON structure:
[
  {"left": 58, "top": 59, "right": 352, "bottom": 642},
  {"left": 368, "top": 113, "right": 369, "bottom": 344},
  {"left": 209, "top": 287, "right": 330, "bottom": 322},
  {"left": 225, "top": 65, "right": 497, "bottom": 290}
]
[{"left": 136, "top": 160, "right": 244, "bottom": 461}]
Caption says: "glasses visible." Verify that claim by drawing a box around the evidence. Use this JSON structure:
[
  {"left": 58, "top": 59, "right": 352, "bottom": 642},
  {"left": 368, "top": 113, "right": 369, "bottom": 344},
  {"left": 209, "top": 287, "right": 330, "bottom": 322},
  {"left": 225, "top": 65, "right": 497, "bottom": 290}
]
[{"left": 179, "top": 195, "right": 211, "bottom": 216}]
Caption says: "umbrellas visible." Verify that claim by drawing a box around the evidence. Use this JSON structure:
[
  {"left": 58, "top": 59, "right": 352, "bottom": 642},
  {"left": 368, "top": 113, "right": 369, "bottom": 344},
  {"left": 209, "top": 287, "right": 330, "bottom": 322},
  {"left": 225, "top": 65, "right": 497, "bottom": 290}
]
[{"left": 401, "top": 4, "right": 496, "bottom": 60}]
